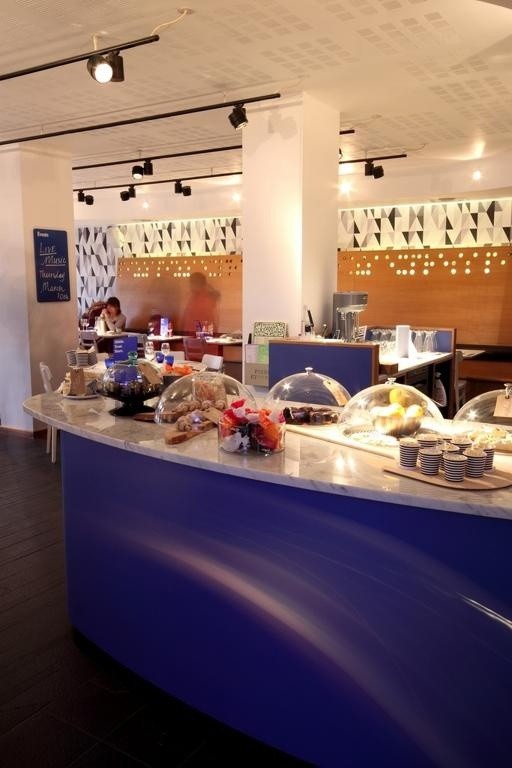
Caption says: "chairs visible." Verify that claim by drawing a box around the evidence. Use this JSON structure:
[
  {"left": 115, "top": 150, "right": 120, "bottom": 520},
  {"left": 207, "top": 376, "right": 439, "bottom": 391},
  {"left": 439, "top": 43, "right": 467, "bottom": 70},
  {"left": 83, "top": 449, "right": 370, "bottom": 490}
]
[
  {"left": 80, "top": 330, "right": 96, "bottom": 349},
  {"left": 39, "top": 361, "right": 60, "bottom": 463},
  {"left": 182, "top": 337, "right": 205, "bottom": 362},
  {"left": 201, "top": 354, "right": 223, "bottom": 371},
  {"left": 155, "top": 349, "right": 185, "bottom": 361}
]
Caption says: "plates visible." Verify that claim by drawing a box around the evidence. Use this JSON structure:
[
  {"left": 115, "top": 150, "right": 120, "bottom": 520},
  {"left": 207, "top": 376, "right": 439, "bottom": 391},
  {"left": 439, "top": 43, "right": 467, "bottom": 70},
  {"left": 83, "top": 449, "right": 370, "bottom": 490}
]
[{"left": 63, "top": 393, "right": 99, "bottom": 400}]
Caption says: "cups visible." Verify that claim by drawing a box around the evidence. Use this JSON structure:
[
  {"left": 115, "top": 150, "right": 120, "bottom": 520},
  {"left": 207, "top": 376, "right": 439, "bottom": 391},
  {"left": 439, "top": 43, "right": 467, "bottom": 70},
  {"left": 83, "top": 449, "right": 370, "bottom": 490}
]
[
  {"left": 399, "top": 437, "right": 495, "bottom": 481},
  {"left": 66, "top": 349, "right": 96, "bottom": 366},
  {"left": 116, "top": 326, "right": 122, "bottom": 334},
  {"left": 371, "top": 328, "right": 437, "bottom": 353}
]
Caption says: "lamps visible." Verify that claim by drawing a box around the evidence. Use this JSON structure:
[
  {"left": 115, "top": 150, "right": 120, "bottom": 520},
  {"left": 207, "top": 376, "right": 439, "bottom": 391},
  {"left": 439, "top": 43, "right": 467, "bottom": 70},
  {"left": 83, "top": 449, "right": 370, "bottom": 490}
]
[
  {"left": 76, "top": 180, "right": 193, "bottom": 205},
  {"left": 229, "top": 103, "right": 249, "bottom": 130},
  {"left": 364, "top": 160, "right": 385, "bottom": 179},
  {"left": 85, "top": 50, "right": 124, "bottom": 83},
  {"left": 132, "top": 159, "right": 154, "bottom": 180}
]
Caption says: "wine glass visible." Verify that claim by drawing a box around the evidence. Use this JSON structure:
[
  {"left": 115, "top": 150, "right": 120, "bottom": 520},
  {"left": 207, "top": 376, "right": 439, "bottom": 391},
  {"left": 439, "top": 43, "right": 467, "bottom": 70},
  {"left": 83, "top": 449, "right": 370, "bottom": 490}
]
[
  {"left": 161, "top": 343, "right": 170, "bottom": 357},
  {"left": 167, "top": 322, "right": 173, "bottom": 338},
  {"left": 81, "top": 319, "right": 91, "bottom": 331},
  {"left": 148, "top": 322, "right": 154, "bottom": 337},
  {"left": 144, "top": 342, "right": 155, "bottom": 363}
]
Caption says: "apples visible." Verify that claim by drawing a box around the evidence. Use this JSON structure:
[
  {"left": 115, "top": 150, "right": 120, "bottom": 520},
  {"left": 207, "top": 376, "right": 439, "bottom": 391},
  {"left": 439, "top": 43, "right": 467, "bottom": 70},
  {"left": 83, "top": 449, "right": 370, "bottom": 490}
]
[{"left": 372, "top": 389, "right": 423, "bottom": 419}]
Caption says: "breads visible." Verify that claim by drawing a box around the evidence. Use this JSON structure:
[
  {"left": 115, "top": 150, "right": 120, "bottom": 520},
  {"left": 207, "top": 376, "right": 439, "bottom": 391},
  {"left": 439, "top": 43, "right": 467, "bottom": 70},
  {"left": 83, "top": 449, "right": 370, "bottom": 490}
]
[
  {"left": 283, "top": 406, "right": 340, "bottom": 425},
  {"left": 174, "top": 400, "right": 227, "bottom": 431}
]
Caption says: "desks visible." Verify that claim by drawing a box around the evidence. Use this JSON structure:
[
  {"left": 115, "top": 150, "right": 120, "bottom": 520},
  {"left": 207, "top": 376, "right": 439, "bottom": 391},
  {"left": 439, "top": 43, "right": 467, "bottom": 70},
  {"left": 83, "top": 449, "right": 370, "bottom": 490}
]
[{"left": 267, "top": 324, "right": 484, "bottom": 419}]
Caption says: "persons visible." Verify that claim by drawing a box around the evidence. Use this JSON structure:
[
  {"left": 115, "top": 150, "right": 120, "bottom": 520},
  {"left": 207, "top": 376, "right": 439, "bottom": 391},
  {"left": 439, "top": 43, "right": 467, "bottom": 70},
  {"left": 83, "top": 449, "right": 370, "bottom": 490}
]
[
  {"left": 181, "top": 272, "right": 217, "bottom": 332},
  {"left": 99, "top": 297, "right": 126, "bottom": 330}
]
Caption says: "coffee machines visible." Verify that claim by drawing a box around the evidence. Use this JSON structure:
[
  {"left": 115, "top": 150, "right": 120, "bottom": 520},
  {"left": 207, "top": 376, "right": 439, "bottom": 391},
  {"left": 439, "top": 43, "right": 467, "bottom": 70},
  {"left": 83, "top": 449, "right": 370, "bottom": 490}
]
[{"left": 333, "top": 293, "right": 368, "bottom": 344}]
